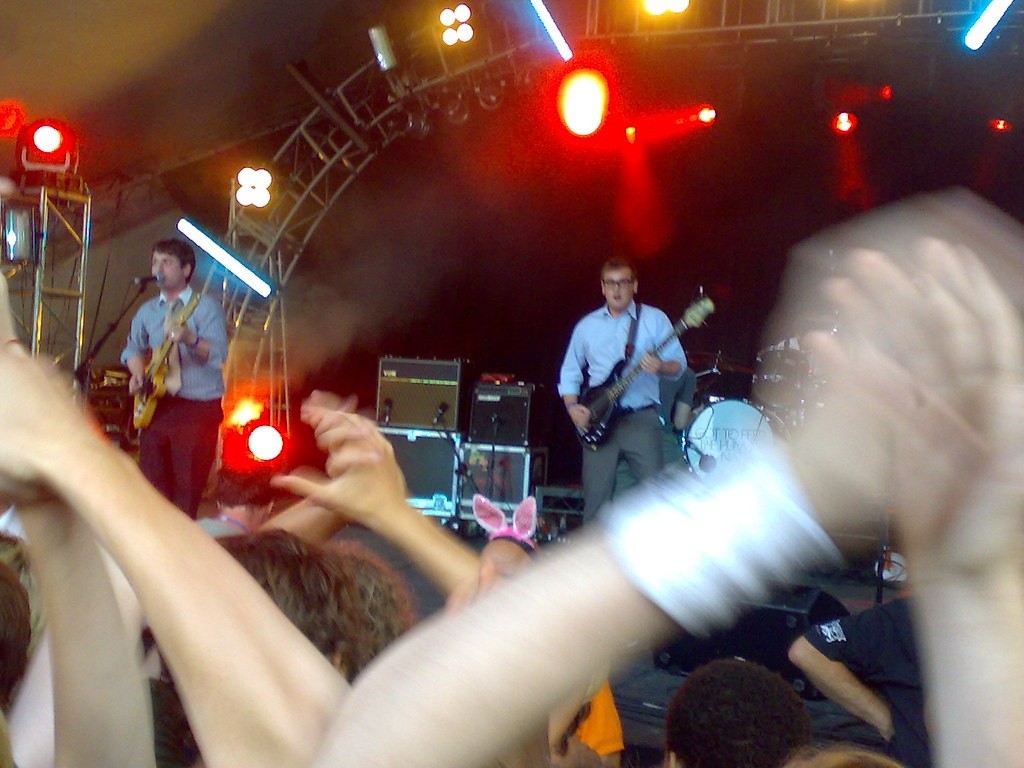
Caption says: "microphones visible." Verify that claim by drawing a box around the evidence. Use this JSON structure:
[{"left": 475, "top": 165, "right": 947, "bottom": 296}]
[
  {"left": 432, "top": 402, "right": 449, "bottom": 424},
  {"left": 384, "top": 398, "right": 393, "bottom": 427},
  {"left": 134, "top": 272, "right": 165, "bottom": 284},
  {"left": 490, "top": 413, "right": 499, "bottom": 422}
]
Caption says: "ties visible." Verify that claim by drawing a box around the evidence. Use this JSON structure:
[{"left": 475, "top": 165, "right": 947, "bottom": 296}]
[{"left": 163, "top": 296, "right": 182, "bottom": 398}]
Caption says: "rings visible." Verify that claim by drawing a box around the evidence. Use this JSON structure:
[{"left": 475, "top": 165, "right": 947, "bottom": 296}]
[{"left": 171, "top": 332, "right": 174, "bottom": 338}]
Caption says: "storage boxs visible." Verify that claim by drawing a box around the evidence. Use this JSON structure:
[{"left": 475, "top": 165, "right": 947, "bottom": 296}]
[{"left": 379, "top": 427, "right": 532, "bottom": 523}]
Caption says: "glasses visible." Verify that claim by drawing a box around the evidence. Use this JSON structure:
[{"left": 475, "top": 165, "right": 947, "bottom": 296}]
[{"left": 603, "top": 279, "right": 633, "bottom": 288}]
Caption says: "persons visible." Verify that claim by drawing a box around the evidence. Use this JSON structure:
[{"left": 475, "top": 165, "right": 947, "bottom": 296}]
[
  {"left": 119, "top": 237, "right": 229, "bottom": 519},
  {"left": 558, "top": 249, "right": 688, "bottom": 526},
  {"left": 0, "top": 196, "right": 1023, "bottom": 768}
]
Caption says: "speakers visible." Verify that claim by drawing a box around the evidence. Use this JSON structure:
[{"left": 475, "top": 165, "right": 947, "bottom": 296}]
[
  {"left": 653, "top": 581, "right": 852, "bottom": 703},
  {"left": 373, "top": 357, "right": 548, "bottom": 446}
]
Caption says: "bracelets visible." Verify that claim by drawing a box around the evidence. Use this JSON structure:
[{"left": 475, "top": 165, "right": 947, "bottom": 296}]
[
  {"left": 188, "top": 335, "right": 199, "bottom": 348},
  {"left": 565, "top": 402, "right": 577, "bottom": 413},
  {"left": 602, "top": 429, "right": 845, "bottom": 636}
]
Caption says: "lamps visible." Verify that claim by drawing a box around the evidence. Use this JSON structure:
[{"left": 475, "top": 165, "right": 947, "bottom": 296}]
[
  {"left": 368, "top": 25, "right": 410, "bottom": 72},
  {"left": 14, "top": 121, "right": 74, "bottom": 173},
  {"left": 0, "top": 201, "right": 39, "bottom": 265}
]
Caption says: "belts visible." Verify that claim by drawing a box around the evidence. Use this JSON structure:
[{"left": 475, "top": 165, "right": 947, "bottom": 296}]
[{"left": 620, "top": 403, "right": 657, "bottom": 415}]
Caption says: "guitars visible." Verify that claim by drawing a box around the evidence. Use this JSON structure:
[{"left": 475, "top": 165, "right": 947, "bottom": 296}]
[
  {"left": 132, "top": 290, "right": 202, "bottom": 430},
  {"left": 576, "top": 296, "right": 716, "bottom": 450}
]
